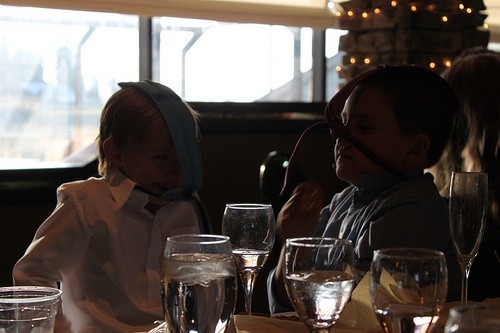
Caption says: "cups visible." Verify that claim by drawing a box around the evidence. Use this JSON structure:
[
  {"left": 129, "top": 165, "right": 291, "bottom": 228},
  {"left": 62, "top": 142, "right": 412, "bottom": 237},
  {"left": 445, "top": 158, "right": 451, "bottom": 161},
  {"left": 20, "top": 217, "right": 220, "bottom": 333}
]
[
  {"left": 444, "top": 302, "right": 500, "bottom": 333},
  {"left": 160, "top": 234, "right": 238, "bottom": 333},
  {"left": 0, "top": 286, "right": 62, "bottom": 333},
  {"left": 369, "top": 248, "right": 449, "bottom": 333}
]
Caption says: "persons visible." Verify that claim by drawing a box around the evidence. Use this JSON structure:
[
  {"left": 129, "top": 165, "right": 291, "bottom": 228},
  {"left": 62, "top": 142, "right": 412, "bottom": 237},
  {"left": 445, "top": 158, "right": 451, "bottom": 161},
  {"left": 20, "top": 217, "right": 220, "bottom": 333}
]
[
  {"left": 423, "top": 54, "right": 500, "bottom": 302},
  {"left": 266, "top": 64, "right": 460, "bottom": 315},
  {"left": 12, "top": 80, "right": 225, "bottom": 333}
]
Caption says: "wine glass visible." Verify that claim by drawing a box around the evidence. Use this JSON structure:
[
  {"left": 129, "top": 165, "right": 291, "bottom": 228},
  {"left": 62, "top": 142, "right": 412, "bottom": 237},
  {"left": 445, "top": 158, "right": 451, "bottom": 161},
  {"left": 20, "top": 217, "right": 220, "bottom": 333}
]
[
  {"left": 448, "top": 171, "right": 489, "bottom": 302},
  {"left": 283, "top": 236, "right": 356, "bottom": 333},
  {"left": 221, "top": 204, "right": 276, "bottom": 316}
]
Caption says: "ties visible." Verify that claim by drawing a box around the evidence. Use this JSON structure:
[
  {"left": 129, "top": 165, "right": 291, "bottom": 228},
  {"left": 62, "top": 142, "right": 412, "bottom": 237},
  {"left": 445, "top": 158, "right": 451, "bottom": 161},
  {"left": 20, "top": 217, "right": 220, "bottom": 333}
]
[
  {"left": 117, "top": 79, "right": 212, "bottom": 233},
  {"left": 279, "top": 67, "right": 415, "bottom": 201}
]
